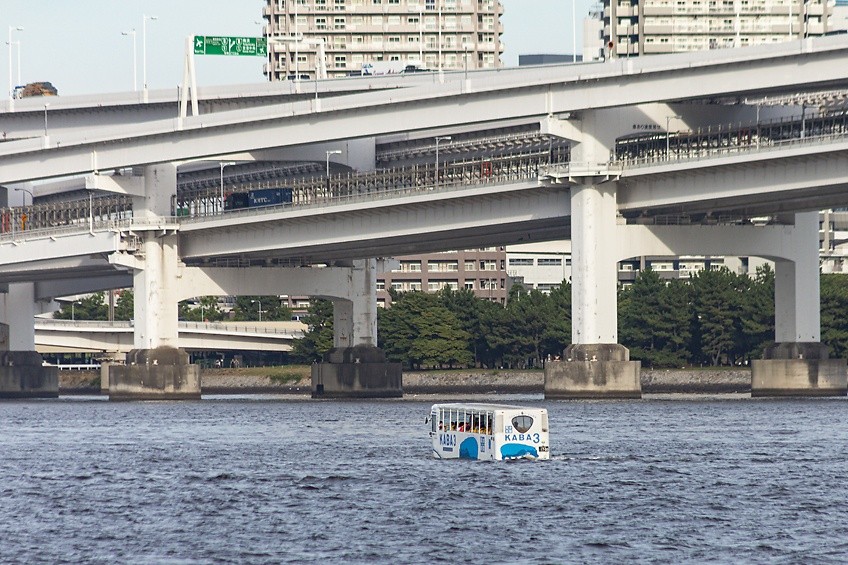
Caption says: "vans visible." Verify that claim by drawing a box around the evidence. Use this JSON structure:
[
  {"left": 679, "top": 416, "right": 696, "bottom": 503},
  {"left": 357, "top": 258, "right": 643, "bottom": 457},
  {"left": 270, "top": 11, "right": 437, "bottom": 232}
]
[{"left": 282, "top": 74, "right": 310, "bottom": 82}]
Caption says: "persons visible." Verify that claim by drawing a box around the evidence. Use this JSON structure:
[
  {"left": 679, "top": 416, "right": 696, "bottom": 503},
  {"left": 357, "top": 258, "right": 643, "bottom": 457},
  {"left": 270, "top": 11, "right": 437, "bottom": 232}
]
[{"left": 440, "top": 414, "right": 521, "bottom": 433}]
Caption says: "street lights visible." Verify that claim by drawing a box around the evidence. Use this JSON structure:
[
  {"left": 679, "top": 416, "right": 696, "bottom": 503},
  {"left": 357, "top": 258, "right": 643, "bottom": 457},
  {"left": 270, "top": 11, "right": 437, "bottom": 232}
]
[
  {"left": 8, "top": 26, "right": 23, "bottom": 112},
  {"left": 665, "top": 115, "right": 682, "bottom": 162},
  {"left": 142, "top": 14, "right": 158, "bottom": 90},
  {"left": 4, "top": 41, "right": 20, "bottom": 86},
  {"left": 121, "top": 28, "right": 138, "bottom": 93},
  {"left": 218, "top": 161, "right": 237, "bottom": 214},
  {"left": 324, "top": 149, "right": 342, "bottom": 202},
  {"left": 14, "top": 189, "right": 34, "bottom": 206},
  {"left": 255, "top": 20, "right": 271, "bottom": 82},
  {"left": 195, "top": 302, "right": 204, "bottom": 322},
  {"left": 251, "top": 300, "right": 262, "bottom": 321},
  {"left": 433, "top": 136, "right": 452, "bottom": 192}
]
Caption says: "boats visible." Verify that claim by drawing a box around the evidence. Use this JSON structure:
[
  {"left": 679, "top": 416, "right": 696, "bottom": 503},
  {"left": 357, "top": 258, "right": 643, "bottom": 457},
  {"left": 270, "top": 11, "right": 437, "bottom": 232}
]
[{"left": 423, "top": 403, "right": 550, "bottom": 462}]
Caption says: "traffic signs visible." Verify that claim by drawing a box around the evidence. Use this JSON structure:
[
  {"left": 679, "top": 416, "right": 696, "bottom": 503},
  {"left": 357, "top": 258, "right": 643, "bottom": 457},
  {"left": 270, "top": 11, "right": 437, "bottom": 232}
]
[{"left": 193, "top": 35, "right": 268, "bottom": 57}]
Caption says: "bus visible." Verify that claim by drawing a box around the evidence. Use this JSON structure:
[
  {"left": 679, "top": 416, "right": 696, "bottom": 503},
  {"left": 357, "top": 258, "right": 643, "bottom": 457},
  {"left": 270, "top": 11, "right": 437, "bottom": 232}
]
[{"left": 360, "top": 59, "right": 425, "bottom": 77}]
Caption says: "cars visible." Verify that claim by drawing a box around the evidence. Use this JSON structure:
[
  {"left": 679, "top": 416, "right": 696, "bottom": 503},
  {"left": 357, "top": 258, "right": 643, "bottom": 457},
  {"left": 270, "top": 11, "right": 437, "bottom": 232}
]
[
  {"left": 30, "top": 82, "right": 57, "bottom": 96},
  {"left": 12, "top": 86, "right": 27, "bottom": 100}
]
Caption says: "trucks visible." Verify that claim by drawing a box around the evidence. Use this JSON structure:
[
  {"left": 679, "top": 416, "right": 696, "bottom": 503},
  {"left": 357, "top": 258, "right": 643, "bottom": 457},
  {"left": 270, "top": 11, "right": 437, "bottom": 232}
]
[{"left": 222, "top": 185, "right": 294, "bottom": 213}]
